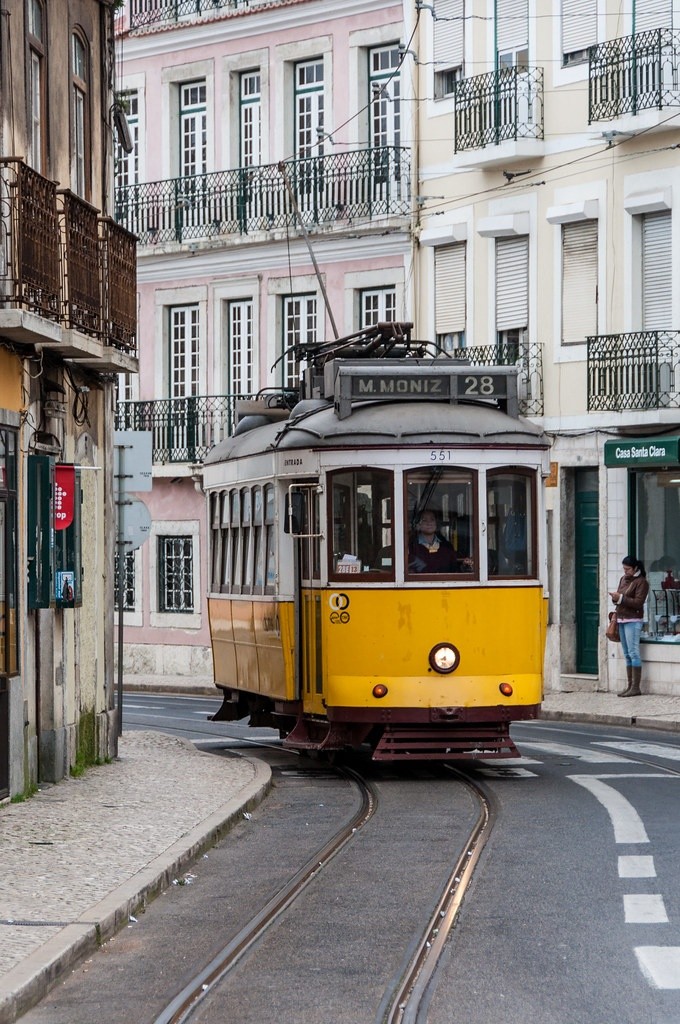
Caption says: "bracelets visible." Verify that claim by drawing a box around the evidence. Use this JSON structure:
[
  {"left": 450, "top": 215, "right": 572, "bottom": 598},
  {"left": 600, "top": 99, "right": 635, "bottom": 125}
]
[{"left": 611, "top": 618, "right": 617, "bottom": 623}]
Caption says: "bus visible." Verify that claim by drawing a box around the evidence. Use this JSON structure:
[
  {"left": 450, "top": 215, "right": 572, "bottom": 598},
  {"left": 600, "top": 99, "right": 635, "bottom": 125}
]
[{"left": 204, "top": 320, "right": 552, "bottom": 757}]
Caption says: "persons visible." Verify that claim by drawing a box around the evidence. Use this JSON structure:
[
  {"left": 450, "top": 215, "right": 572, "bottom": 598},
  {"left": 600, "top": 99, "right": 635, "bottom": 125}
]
[
  {"left": 608, "top": 555, "right": 649, "bottom": 697},
  {"left": 408, "top": 509, "right": 475, "bottom": 573}
]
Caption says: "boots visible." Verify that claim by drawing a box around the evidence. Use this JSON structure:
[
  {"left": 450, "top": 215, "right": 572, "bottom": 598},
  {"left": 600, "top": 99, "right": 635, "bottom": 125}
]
[
  {"left": 621, "top": 666, "right": 641, "bottom": 697},
  {"left": 618, "top": 665, "right": 631, "bottom": 696}
]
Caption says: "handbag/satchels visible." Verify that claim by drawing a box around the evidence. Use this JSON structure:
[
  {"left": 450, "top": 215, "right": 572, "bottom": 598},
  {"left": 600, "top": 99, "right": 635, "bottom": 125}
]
[{"left": 606, "top": 612, "right": 620, "bottom": 642}]
